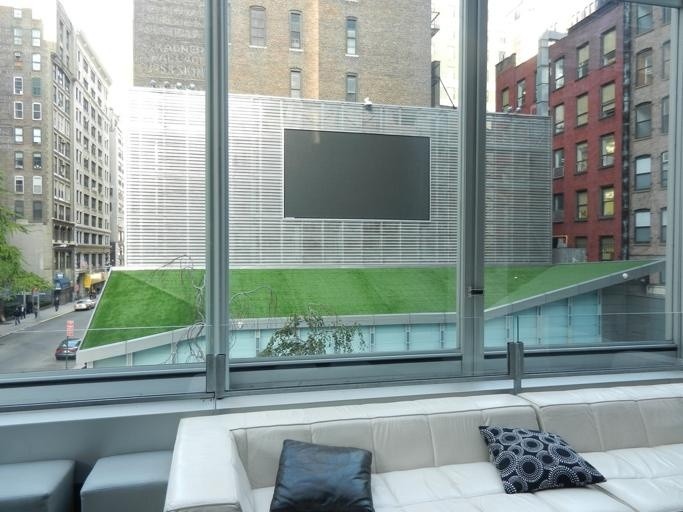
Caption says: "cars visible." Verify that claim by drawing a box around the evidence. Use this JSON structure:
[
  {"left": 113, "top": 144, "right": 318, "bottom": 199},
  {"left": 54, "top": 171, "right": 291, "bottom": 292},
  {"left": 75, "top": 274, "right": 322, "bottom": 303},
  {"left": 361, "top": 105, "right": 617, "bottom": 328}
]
[
  {"left": 56, "top": 338, "right": 81, "bottom": 360},
  {"left": 75, "top": 300, "right": 96, "bottom": 310}
]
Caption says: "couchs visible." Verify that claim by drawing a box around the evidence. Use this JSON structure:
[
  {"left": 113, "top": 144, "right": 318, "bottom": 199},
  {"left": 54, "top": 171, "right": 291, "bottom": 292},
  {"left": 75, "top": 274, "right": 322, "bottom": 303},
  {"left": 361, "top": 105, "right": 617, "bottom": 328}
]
[{"left": 163, "top": 380, "right": 681, "bottom": 511}]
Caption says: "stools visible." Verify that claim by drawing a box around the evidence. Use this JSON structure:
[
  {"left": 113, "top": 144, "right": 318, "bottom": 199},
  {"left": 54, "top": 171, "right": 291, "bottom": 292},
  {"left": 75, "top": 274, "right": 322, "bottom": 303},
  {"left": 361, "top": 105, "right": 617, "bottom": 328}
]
[{"left": 0, "top": 446, "right": 173, "bottom": 510}]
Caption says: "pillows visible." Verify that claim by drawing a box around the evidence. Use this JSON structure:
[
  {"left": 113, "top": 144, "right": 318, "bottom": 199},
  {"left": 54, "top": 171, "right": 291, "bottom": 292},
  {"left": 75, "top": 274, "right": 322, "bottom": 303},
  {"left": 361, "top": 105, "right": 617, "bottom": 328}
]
[
  {"left": 477, "top": 422, "right": 608, "bottom": 493},
  {"left": 270, "top": 439, "right": 376, "bottom": 512}
]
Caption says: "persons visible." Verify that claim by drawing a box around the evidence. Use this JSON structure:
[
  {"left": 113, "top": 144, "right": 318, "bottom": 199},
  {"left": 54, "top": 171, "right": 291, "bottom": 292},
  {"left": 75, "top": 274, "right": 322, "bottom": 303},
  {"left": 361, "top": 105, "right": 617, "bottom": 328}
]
[
  {"left": 14, "top": 307, "right": 21, "bottom": 326},
  {"left": 32, "top": 301, "right": 39, "bottom": 318},
  {"left": 54, "top": 295, "right": 60, "bottom": 311},
  {"left": 19, "top": 302, "right": 26, "bottom": 321}
]
